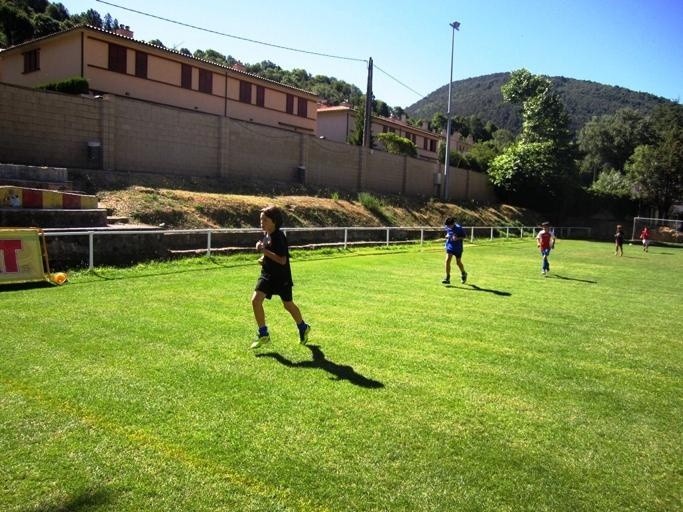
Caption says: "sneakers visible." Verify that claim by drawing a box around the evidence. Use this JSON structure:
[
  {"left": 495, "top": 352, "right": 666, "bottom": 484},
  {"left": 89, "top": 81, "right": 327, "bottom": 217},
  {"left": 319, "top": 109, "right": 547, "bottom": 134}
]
[
  {"left": 442, "top": 279, "right": 450, "bottom": 284},
  {"left": 461, "top": 273, "right": 467, "bottom": 283},
  {"left": 250, "top": 334, "right": 270, "bottom": 348},
  {"left": 301, "top": 324, "right": 310, "bottom": 344}
]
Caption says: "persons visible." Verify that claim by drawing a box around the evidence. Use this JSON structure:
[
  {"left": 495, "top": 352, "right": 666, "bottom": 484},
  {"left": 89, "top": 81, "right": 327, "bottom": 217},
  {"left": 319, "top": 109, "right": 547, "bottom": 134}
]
[
  {"left": 441, "top": 216, "right": 468, "bottom": 284},
  {"left": 536, "top": 221, "right": 556, "bottom": 275},
  {"left": 614, "top": 224, "right": 624, "bottom": 257},
  {"left": 639, "top": 225, "right": 651, "bottom": 252},
  {"left": 247, "top": 206, "right": 311, "bottom": 350}
]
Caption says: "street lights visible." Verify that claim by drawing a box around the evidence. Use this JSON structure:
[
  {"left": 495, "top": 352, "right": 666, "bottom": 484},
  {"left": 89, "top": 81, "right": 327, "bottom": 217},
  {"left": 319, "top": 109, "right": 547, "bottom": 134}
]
[{"left": 442, "top": 19, "right": 461, "bottom": 201}]
